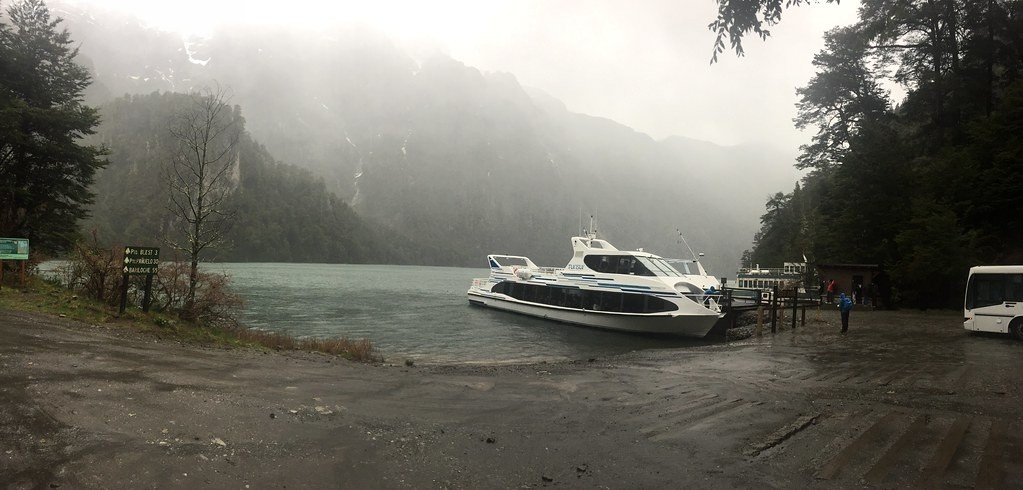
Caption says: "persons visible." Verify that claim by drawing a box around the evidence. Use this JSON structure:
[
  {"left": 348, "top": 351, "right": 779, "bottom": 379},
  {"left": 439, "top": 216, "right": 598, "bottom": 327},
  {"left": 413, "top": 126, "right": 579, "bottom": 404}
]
[
  {"left": 600, "top": 260, "right": 629, "bottom": 273},
  {"left": 819, "top": 279, "right": 835, "bottom": 305},
  {"left": 837, "top": 293, "right": 850, "bottom": 334},
  {"left": 850, "top": 279, "right": 878, "bottom": 308},
  {"left": 703, "top": 286, "right": 720, "bottom": 309},
  {"left": 545, "top": 293, "right": 610, "bottom": 310}
]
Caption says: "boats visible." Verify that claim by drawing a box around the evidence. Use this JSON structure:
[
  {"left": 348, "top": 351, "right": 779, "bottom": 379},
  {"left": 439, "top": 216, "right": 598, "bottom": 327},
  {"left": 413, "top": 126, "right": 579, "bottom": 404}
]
[
  {"left": 721, "top": 261, "right": 821, "bottom": 292},
  {"left": 468, "top": 207, "right": 771, "bottom": 339}
]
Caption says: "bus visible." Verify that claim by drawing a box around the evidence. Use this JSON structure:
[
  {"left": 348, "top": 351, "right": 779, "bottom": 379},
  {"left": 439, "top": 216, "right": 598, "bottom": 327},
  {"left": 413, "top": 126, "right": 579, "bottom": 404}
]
[{"left": 963, "top": 266, "right": 1023, "bottom": 340}]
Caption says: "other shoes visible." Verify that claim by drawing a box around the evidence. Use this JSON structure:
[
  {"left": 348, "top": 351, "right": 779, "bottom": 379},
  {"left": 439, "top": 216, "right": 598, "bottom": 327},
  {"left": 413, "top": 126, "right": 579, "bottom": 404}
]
[{"left": 825, "top": 302, "right": 831, "bottom": 304}]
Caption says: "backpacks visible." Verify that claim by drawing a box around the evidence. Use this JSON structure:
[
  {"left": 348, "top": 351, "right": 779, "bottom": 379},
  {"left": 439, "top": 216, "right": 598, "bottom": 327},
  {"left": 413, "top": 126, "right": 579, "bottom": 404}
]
[{"left": 843, "top": 299, "right": 852, "bottom": 310}]
